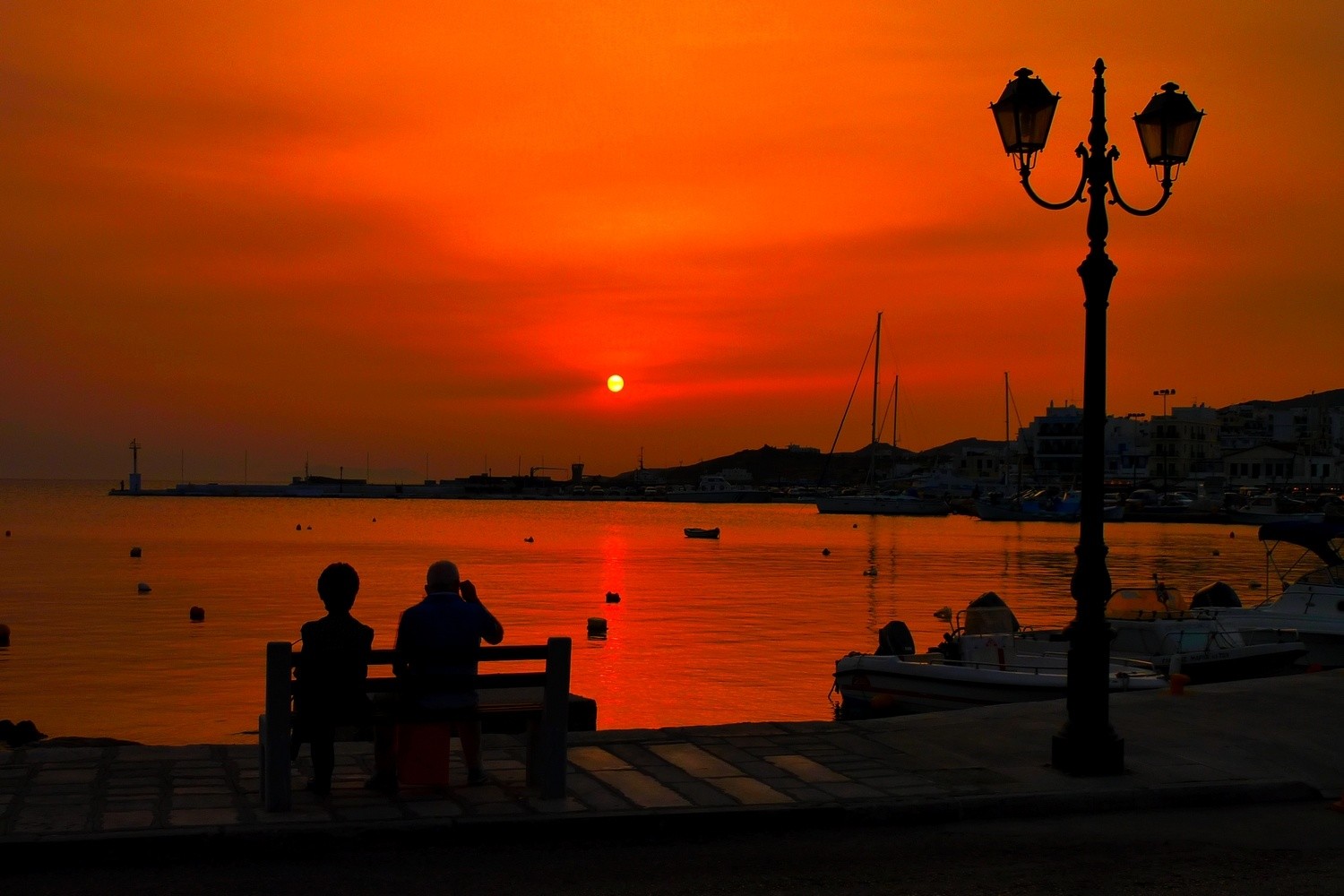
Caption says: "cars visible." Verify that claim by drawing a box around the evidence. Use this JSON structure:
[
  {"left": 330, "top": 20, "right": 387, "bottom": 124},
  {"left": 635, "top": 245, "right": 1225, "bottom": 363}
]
[
  {"left": 572, "top": 485, "right": 586, "bottom": 495},
  {"left": 608, "top": 486, "right": 620, "bottom": 496},
  {"left": 588, "top": 485, "right": 604, "bottom": 495},
  {"left": 644, "top": 486, "right": 659, "bottom": 496},
  {"left": 624, "top": 486, "right": 638, "bottom": 496}
]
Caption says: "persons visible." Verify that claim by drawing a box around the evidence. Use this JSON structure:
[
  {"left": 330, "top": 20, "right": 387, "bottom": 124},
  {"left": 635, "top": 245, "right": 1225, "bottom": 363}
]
[{"left": 289, "top": 560, "right": 503, "bottom": 799}]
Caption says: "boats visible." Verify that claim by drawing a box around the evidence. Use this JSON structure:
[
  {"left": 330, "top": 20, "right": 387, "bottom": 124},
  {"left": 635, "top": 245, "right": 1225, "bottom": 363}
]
[
  {"left": 684, "top": 526, "right": 719, "bottom": 539},
  {"left": 666, "top": 476, "right": 776, "bottom": 503},
  {"left": 809, "top": 308, "right": 1344, "bottom": 725}
]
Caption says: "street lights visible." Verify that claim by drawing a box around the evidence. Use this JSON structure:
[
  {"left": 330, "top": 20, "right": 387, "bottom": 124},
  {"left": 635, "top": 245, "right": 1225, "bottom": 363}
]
[
  {"left": 1152, "top": 388, "right": 1176, "bottom": 506},
  {"left": 989, "top": 54, "right": 1211, "bottom": 784}
]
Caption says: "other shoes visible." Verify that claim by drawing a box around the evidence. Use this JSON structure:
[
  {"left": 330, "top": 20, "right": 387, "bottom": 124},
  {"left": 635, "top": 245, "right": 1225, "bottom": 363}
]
[
  {"left": 308, "top": 775, "right": 332, "bottom": 791},
  {"left": 363, "top": 772, "right": 398, "bottom": 791},
  {"left": 469, "top": 770, "right": 498, "bottom": 786}
]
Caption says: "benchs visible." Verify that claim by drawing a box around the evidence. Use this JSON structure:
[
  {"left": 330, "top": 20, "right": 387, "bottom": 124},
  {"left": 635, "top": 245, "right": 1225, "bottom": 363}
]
[{"left": 257, "top": 636, "right": 573, "bottom": 813}]
